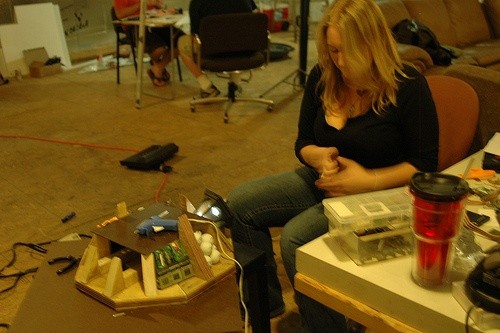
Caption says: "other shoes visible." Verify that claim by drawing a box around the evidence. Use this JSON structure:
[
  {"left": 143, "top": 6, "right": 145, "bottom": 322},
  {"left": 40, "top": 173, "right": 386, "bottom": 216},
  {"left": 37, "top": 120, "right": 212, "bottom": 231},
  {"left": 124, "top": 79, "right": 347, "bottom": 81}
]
[{"left": 193, "top": 84, "right": 220, "bottom": 100}]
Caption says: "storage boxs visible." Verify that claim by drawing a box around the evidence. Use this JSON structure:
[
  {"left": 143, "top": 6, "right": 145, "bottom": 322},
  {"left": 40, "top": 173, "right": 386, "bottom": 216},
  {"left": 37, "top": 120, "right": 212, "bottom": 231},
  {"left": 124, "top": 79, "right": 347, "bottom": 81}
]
[
  {"left": 23, "top": 47, "right": 65, "bottom": 78},
  {"left": 256, "top": 3, "right": 290, "bottom": 32},
  {"left": 322, "top": 185, "right": 415, "bottom": 266}
]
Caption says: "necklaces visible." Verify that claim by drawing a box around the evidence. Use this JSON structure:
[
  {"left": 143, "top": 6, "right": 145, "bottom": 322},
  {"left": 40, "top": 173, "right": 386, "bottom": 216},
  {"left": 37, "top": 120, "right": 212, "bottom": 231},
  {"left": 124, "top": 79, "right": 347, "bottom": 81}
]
[{"left": 347, "top": 97, "right": 360, "bottom": 117}]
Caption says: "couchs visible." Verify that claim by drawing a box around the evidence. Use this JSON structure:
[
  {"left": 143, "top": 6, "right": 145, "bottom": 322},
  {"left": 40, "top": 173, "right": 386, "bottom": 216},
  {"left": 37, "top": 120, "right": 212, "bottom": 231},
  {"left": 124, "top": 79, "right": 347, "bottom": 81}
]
[{"left": 376, "top": 0, "right": 500, "bottom": 78}]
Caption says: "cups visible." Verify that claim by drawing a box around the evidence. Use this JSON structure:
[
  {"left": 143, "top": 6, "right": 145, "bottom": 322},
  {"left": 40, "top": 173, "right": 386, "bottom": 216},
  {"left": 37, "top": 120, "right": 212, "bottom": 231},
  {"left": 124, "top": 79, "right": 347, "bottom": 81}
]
[{"left": 410, "top": 171, "right": 470, "bottom": 291}]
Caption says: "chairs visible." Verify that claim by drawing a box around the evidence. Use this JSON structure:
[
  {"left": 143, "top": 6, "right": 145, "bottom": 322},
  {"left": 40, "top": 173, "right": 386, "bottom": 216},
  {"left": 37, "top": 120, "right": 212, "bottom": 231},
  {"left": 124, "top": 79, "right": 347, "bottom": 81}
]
[
  {"left": 426, "top": 74, "right": 480, "bottom": 172},
  {"left": 111, "top": 7, "right": 182, "bottom": 85},
  {"left": 190, "top": 13, "right": 275, "bottom": 123}
]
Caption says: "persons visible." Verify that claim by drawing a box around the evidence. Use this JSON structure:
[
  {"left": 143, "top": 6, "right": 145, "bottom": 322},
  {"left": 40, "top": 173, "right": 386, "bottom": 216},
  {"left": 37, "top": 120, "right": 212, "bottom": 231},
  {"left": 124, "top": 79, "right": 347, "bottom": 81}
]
[
  {"left": 178, "top": 0, "right": 257, "bottom": 100},
  {"left": 114, "top": 0, "right": 179, "bottom": 86},
  {"left": 227, "top": 0, "right": 439, "bottom": 333}
]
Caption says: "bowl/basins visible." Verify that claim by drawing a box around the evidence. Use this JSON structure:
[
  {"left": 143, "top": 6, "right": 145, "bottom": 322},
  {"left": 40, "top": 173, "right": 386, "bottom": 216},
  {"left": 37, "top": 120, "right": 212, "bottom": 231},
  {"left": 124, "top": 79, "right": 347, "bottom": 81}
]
[{"left": 263, "top": 43, "right": 295, "bottom": 60}]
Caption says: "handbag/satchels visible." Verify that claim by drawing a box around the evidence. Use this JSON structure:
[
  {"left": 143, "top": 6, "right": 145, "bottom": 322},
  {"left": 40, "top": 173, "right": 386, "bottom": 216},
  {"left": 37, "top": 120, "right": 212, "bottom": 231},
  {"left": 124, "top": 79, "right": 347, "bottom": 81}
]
[{"left": 392, "top": 19, "right": 458, "bottom": 66}]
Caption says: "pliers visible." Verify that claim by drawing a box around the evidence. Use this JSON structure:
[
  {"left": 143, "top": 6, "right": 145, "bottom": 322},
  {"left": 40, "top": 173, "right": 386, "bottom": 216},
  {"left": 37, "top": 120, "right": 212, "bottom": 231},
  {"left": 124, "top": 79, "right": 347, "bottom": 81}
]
[{"left": 48, "top": 254, "right": 83, "bottom": 275}]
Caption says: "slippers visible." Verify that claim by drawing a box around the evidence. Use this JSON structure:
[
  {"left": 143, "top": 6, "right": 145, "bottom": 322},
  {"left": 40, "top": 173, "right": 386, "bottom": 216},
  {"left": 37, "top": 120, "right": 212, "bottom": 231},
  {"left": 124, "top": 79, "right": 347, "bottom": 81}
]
[
  {"left": 148, "top": 69, "right": 164, "bottom": 85},
  {"left": 162, "top": 70, "right": 170, "bottom": 81}
]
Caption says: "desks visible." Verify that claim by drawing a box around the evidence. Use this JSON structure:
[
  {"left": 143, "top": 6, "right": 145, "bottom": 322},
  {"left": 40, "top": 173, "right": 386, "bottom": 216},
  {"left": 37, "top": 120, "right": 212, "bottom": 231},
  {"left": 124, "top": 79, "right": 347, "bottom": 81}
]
[
  {"left": 112, "top": 10, "right": 189, "bottom": 93},
  {"left": 6, "top": 202, "right": 243, "bottom": 333},
  {"left": 294, "top": 150, "right": 500, "bottom": 333}
]
